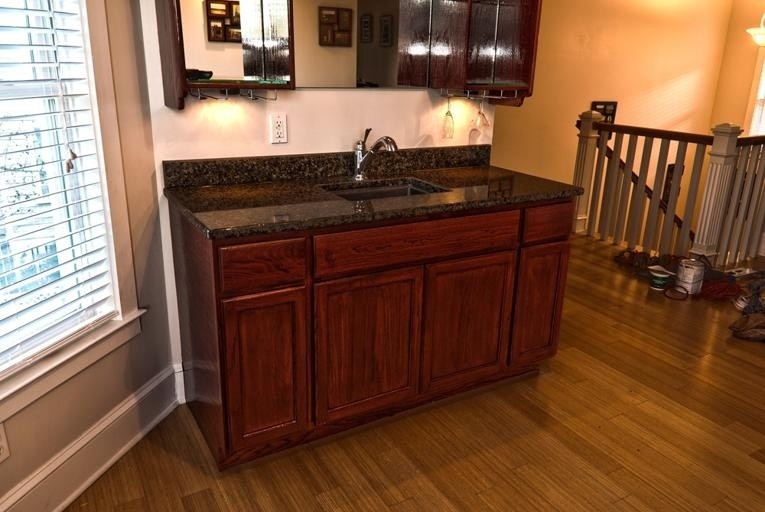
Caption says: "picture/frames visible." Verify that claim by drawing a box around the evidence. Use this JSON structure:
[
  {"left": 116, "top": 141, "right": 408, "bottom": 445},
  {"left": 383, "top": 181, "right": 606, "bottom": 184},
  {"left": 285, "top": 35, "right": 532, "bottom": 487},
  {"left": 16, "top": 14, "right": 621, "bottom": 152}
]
[
  {"left": 359, "top": 15, "right": 373, "bottom": 43},
  {"left": 379, "top": 15, "right": 393, "bottom": 48}
]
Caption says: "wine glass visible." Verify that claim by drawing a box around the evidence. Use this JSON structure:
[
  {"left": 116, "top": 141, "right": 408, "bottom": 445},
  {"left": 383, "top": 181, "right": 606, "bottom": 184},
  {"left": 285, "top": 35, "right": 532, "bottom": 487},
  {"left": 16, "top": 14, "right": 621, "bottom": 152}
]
[
  {"left": 470, "top": 99, "right": 492, "bottom": 130},
  {"left": 442, "top": 97, "right": 455, "bottom": 139}
]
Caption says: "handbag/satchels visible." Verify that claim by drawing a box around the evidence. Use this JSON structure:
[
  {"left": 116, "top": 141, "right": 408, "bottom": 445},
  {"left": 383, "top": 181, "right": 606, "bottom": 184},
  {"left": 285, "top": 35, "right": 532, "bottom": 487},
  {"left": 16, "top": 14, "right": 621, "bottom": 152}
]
[{"left": 729, "top": 313, "right": 765, "bottom": 341}]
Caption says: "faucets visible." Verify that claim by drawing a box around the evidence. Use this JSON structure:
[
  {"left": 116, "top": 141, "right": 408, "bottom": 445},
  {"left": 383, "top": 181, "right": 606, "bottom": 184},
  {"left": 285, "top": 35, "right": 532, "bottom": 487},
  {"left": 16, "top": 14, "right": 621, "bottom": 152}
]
[{"left": 352, "top": 127, "right": 398, "bottom": 181}]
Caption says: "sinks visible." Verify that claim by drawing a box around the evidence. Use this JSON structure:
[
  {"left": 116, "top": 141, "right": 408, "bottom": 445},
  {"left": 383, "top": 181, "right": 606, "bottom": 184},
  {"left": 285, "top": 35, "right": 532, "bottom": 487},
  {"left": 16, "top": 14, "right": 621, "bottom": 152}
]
[{"left": 315, "top": 176, "right": 454, "bottom": 202}]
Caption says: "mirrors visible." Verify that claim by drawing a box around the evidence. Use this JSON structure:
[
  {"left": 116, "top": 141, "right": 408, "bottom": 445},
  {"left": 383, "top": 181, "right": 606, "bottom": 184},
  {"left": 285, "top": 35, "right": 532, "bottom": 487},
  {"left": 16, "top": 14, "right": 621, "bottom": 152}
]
[{"left": 178, "top": 0, "right": 537, "bottom": 89}]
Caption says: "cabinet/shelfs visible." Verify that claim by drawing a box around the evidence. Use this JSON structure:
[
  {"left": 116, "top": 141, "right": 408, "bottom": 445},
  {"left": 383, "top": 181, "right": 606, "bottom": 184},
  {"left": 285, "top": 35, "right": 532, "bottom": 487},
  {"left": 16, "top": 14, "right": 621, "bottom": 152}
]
[
  {"left": 396, "top": 0, "right": 470, "bottom": 88},
  {"left": 465, "top": 0, "right": 533, "bottom": 87},
  {"left": 169, "top": 203, "right": 311, "bottom": 473},
  {"left": 311, "top": 202, "right": 523, "bottom": 441},
  {"left": 509, "top": 194, "right": 578, "bottom": 381}
]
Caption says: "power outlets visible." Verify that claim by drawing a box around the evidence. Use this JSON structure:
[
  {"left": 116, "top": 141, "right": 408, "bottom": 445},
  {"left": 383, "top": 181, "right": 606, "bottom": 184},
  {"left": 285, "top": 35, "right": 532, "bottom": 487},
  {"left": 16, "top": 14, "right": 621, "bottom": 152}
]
[{"left": 269, "top": 111, "right": 287, "bottom": 143}]
